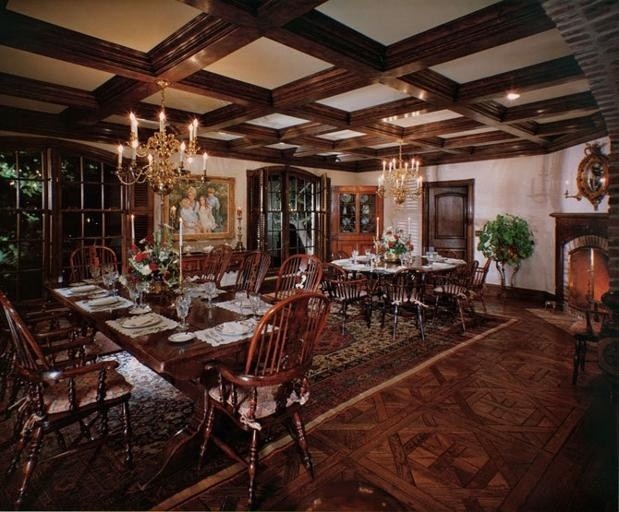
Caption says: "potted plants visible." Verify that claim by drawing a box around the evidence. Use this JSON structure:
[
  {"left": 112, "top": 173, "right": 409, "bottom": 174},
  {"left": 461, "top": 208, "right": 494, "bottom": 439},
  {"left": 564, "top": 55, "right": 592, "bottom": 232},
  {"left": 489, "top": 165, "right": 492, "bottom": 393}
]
[{"left": 475, "top": 212, "right": 536, "bottom": 267}]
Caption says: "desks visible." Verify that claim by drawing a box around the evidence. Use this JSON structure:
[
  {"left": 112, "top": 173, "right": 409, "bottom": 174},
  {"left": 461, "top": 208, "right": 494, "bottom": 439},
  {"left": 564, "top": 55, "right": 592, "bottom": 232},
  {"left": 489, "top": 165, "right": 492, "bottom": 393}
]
[
  {"left": 595, "top": 337, "right": 619, "bottom": 408},
  {"left": 494, "top": 259, "right": 521, "bottom": 301}
]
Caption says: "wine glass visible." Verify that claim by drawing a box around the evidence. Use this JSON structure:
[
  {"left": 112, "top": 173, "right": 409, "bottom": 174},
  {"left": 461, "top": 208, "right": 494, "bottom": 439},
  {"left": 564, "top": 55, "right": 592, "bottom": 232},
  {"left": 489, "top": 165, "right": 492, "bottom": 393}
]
[
  {"left": 235, "top": 290, "right": 247, "bottom": 314},
  {"left": 399, "top": 252, "right": 414, "bottom": 268},
  {"left": 175, "top": 296, "right": 191, "bottom": 331},
  {"left": 204, "top": 282, "right": 216, "bottom": 309},
  {"left": 351, "top": 249, "right": 358, "bottom": 264},
  {"left": 129, "top": 280, "right": 148, "bottom": 308},
  {"left": 365, "top": 249, "right": 371, "bottom": 266},
  {"left": 102, "top": 270, "right": 119, "bottom": 295},
  {"left": 249, "top": 291, "right": 261, "bottom": 320},
  {"left": 426, "top": 252, "right": 438, "bottom": 266}
]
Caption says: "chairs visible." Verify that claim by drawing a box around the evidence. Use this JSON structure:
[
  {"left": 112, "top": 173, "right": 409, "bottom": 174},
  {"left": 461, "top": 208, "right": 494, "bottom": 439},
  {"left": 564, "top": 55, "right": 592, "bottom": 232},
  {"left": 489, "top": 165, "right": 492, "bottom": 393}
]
[{"left": 565, "top": 288, "right": 619, "bottom": 385}]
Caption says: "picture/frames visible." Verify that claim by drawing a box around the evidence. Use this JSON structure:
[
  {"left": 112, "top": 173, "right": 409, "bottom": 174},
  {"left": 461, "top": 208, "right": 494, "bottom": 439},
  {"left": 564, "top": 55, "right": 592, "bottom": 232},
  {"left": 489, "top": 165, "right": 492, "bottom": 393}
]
[{"left": 158, "top": 174, "right": 236, "bottom": 239}]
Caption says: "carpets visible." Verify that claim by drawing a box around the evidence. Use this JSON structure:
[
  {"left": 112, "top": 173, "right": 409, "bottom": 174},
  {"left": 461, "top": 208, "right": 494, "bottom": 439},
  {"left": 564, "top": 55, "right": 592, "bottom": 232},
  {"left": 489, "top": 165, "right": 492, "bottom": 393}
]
[{"left": 525, "top": 306, "right": 588, "bottom": 336}]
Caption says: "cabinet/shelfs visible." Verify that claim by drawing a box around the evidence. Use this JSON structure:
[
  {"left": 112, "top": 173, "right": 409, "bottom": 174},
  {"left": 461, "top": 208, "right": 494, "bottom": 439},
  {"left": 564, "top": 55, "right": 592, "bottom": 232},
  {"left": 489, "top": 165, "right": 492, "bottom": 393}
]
[{"left": 329, "top": 184, "right": 384, "bottom": 241}]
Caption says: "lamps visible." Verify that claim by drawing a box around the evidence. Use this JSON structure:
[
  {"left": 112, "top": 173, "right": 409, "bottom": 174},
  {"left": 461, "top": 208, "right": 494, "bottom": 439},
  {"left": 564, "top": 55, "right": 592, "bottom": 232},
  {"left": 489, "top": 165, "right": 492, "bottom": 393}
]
[
  {"left": 373, "top": 139, "right": 425, "bottom": 207},
  {"left": 112, "top": 77, "right": 209, "bottom": 202}
]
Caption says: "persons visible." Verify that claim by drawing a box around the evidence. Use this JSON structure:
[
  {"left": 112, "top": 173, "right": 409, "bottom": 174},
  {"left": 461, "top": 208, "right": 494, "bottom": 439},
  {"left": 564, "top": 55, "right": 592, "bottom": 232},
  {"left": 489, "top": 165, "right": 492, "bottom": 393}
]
[
  {"left": 197, "top": 194, "right": 217, "bottom": 236},
  {"left": 206, "top": 186, "right": 220, "bottom": 216},
  {"left": 175, "top": 186, "right": 200, "bottom": 236},
  {"left": 179, "top": 199, "right": 200, "bottom": 235}
]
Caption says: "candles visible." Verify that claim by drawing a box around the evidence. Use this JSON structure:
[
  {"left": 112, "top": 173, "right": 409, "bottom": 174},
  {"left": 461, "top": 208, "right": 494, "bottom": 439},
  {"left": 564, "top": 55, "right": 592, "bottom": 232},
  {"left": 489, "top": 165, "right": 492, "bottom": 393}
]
[
  {"left": 407, "top": 216, "right": 412, "bottom": 234},
  {"left": 236, "top": 206, "right": 244, "bottom": 218},
  {"left": 177, "top": 217, "right": 184, "bottom": 246},
  {"left": 129, "top": 214, "right": 137, "bottom": 241},
  {"left": 376, "top": 216, "right": 380, "bottom": 237}
]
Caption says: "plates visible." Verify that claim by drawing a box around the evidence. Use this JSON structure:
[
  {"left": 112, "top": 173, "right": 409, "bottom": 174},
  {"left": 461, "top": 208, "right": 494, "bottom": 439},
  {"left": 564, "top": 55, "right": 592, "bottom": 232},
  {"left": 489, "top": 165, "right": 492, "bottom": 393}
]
[
  {"left": 168, "top": 332, "right": 195, "bottom": 342},
  {"left": 120, "top": 315, "right": 161, "bottom": 328},
  {"left": 215, "top": 320, "right": 255, "bottom": 335},
  {"left": 70, "top": 285, "right": 97, "bottom": 292},
  {"left": 89, "top": 298, "right": 118, "bottom": 305},
  {"left": 235, "top": 301, "right": 264, "bottom": 307}
]
